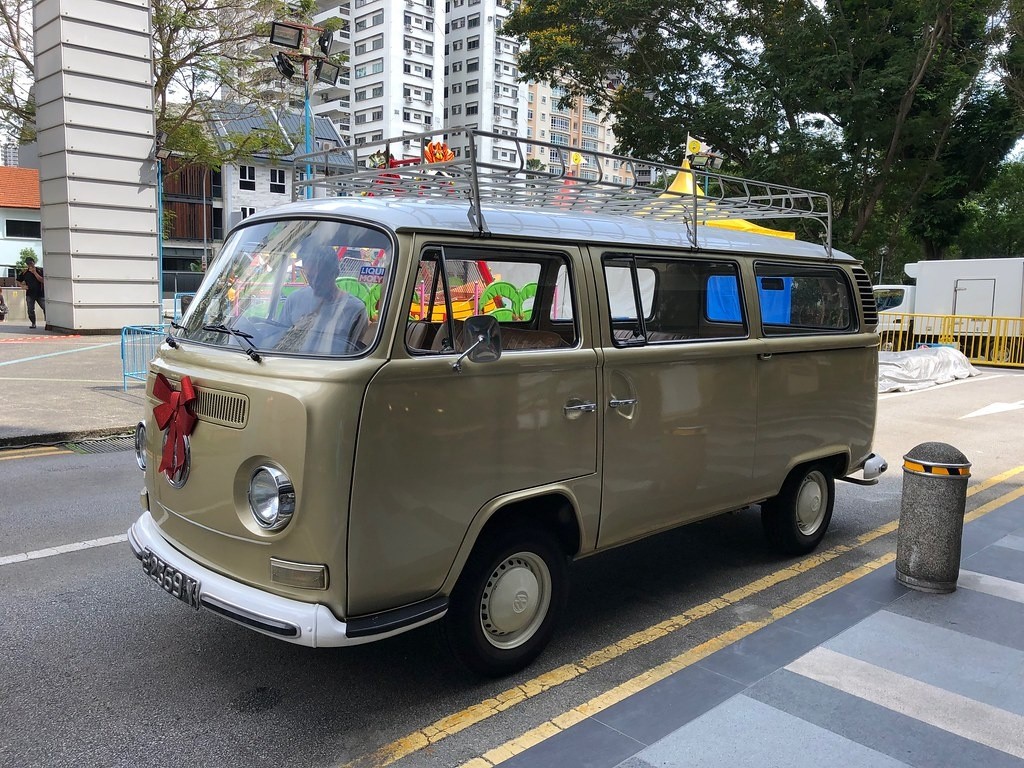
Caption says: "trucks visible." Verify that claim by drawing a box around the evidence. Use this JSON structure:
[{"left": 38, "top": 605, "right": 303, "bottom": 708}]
[{"left": 869, "top": 256, "right": 1024, "bottom": 369}]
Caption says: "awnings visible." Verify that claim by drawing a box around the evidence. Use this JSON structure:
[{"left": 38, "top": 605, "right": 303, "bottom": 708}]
[
  {"left": 596, "top": 157, "right": 796, "bottom": 328},
  {"left": 518, "top": 170, "right": 594, "bottom": 222}
]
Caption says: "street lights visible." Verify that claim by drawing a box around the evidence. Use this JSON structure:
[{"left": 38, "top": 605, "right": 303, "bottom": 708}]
[{"left": 269, "top": 19, "right": 342, "bottom": 201}]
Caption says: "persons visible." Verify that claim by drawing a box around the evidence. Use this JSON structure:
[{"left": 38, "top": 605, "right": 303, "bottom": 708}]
[
  {"left": 279, "top": 243, "right": 370, "bottom": 339},
  {"left": 14, "top": 257, "right": 44, "bottom": 328}
]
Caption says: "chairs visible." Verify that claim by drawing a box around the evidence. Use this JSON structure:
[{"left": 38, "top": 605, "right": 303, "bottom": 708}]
[{"left": 358, "top": 320, "right": 695, "bottom": 353}]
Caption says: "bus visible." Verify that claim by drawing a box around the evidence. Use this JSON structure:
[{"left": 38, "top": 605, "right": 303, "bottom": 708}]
[{"left": 126, "top": 125, "right": 890, "bottom": 685}]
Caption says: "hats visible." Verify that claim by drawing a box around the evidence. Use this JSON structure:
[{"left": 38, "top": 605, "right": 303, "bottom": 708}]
[{"left": 26, "top": 257, "right": 34, "bottom": 264}]
